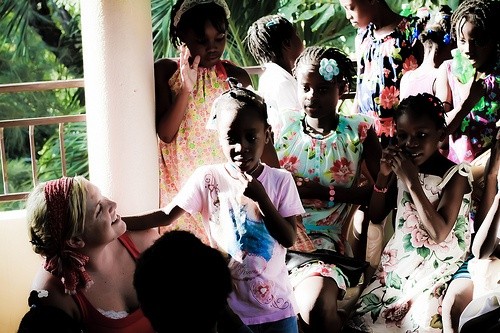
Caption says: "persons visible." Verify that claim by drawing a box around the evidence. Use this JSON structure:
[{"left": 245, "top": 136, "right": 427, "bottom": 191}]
[
  {"left": 439, "top": 128, "right": 500, "bottom": 333},
  {"left": 16, "top": 177, "right": 161, "bottom": 333},
  {"left": 134, "top": 229, "right": 251, "bottom": 332},
  {"left": 117, "top": 79, "right": 306, "bottom": 333},
  {"left": 338, "top": 0, "right": 419, "bottom": 136},
  {"left": 154, "top": 0, "right": 254, "bottom": 248},
  {"left": 435, "top": 0, "right": 500, "bottom": 189},
  {"left": 248, "top": 15, "right": 306, "bottom": 146},
  {"left": 273, "top": 46, "right": 376, "bottom": 333},
  {"left": 343, "top": 93, "right": 472, "bottom": 333},
  {"left": 397, "top": 6, "right": 455, "bottom": 103},
  {"left": 468, "top": 191, "right": 500, "bottom": 260}
]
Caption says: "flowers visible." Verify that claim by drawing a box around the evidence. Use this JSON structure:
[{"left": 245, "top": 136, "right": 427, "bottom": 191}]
[
  {"left": 368, "top": 55, "right": 418, "bottom": 136},
  {"left": 319, "top": 58, "right": 339, "bottom": 81}
]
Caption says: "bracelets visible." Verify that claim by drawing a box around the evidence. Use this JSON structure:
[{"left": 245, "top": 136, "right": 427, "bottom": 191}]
[
  {"left": 296, "top": 175, "right": 312, "bottom": 190},
  {"left": 327, "top": 185, "right": 337, "bottom": 211}
]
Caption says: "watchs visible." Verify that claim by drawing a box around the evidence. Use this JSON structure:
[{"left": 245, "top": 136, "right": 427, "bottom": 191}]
[
  {"left": 181, "top": 86, "right": 194, "bottom": 96},
  {"left": 376, "top": 185, "right": 389, "bottom": 195}
]
